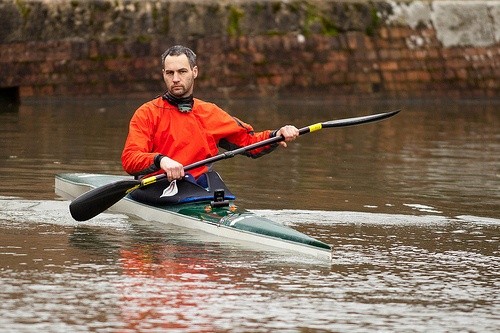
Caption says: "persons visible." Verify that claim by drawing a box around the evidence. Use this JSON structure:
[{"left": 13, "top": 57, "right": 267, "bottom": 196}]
[{"left": 121, "top": 45, "right": 299, "bottom": 182}]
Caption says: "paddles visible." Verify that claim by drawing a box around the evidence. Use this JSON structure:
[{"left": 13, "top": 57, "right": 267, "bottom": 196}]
[{"left": 69, "top": 108, "right": 402, "bottom": 223}]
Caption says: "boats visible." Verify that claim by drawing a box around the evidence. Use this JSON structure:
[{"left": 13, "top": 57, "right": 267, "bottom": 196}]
[{"left": 55, "top": 173, "right": 334, "bottom": 267}]
